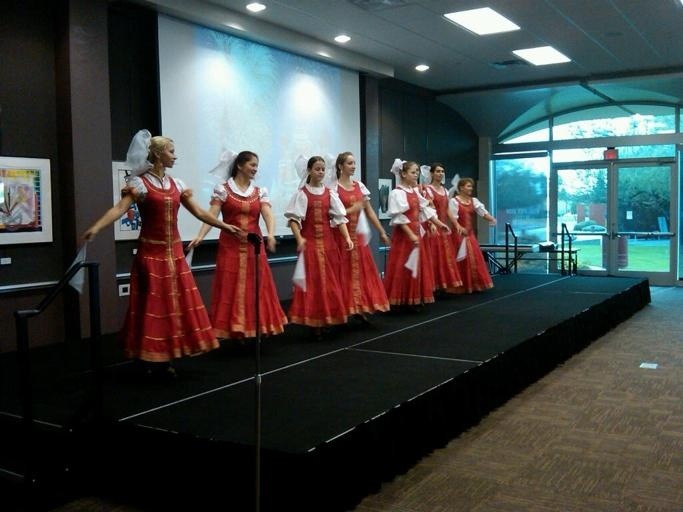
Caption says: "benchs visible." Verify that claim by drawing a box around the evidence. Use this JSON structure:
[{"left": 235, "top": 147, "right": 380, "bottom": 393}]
[{"left": 480, "top": 242, "right": 581, "bottom": 278}]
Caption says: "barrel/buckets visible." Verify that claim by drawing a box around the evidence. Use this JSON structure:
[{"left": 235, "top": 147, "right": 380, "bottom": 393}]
[{"left": 602, "top": 234, "right": 628, "bottom": 267}]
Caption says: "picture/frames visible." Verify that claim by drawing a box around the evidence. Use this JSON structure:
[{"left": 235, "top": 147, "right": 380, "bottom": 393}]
[
  {"left": 377, "top": 177, "right": 394, "bottom": 220},
  {"left": 112, "top": 161, "right": 143, "bottom": 241},
  {"left": 0, "top": 157, "right": 54, "bottom": 245}
]
